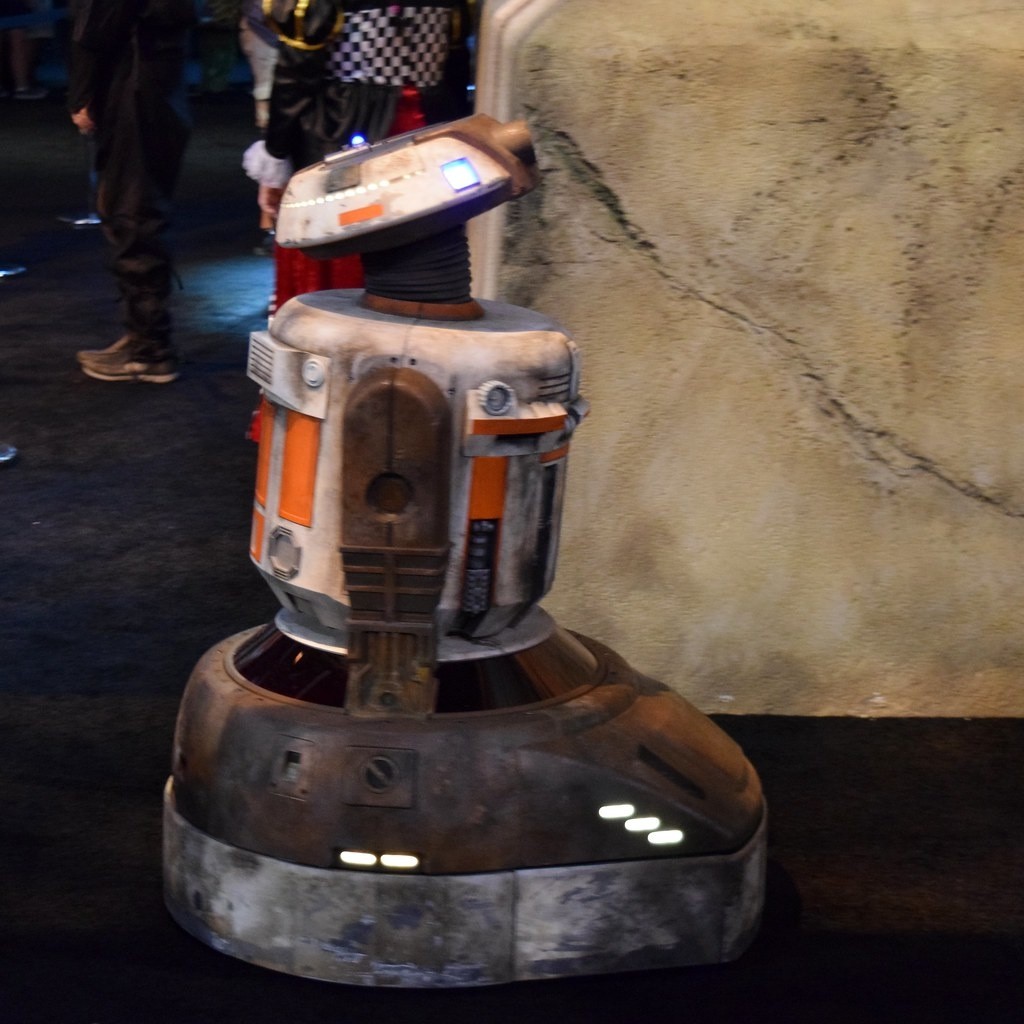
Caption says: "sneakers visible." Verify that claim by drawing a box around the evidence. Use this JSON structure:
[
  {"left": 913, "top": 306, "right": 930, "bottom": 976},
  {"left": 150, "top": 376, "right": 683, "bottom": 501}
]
[
  {"left": 75, "top": 332, "right": 133, "bottom": 363},
  {"left": 82, "top": 343, "right": 179, "bottom": 381}
]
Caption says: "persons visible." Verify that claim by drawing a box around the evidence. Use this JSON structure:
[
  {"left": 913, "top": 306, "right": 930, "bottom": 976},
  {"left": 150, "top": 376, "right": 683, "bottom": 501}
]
[
  {"left": 0, "top": 0, "right": 47, "bottom": 98},
  {"left": 240, "top": 0, "right": 472, "bottom": 317},
  {"left": 57, "top": 0, "right": 190, "bottom": 385}
]
